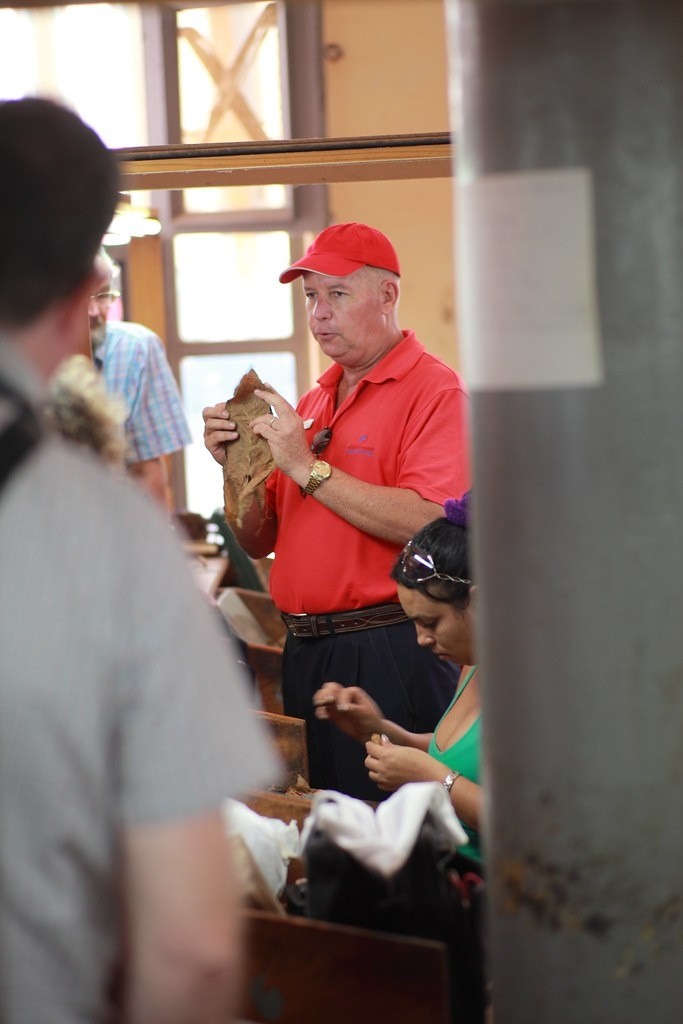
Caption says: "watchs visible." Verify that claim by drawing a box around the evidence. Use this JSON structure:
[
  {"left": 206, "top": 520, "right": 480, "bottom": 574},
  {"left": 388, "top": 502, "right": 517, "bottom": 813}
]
[{"left": 304, "top": 460, "right": 332, "bottom": 495}]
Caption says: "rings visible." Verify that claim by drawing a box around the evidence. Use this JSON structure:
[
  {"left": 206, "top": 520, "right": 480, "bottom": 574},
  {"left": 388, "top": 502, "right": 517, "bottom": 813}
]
[{"left": 269, "top": 416, "right": 276, "bottom": 427}]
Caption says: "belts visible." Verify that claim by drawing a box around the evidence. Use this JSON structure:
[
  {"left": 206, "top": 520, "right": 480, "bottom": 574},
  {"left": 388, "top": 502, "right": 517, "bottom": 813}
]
[{"left": 280, "top": 603, "right": 412, "bottom": 637}]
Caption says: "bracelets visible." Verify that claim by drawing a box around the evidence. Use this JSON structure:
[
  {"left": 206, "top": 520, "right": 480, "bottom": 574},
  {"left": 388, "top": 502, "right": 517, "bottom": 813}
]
[{"left": 443, "top": 769, "right": 461, "bottom": 793}]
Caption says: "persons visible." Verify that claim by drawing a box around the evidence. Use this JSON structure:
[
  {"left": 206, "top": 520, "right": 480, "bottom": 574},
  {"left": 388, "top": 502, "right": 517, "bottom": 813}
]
[
  {"left": 88, "top": 248, "right": 193, "bottom": 525},
  {"left": 202, "top": 222, "right": 484, "bottom": 1024},
  {"left": 315, "top": 478, "right": 483, "bottom": 1024},
  {"left": 0, "top": 98, "right": 289, "bottom": 1024}
]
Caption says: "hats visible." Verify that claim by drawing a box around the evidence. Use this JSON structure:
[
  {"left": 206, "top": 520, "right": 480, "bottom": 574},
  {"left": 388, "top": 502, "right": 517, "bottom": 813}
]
[{"left": 278, "top": 222, "right": 400, "bottom": 281}]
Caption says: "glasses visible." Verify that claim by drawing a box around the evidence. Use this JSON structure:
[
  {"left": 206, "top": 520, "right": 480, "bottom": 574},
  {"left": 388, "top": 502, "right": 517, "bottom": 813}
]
[
  {"left": 307, "top": 426, "right": 332, "bottom": 458},
  {"left": 399, "top": 541, "right": 473, "bottom": 594},
  {"left": 88, "top": 290, "right": 121, "bottom": 303}
]
